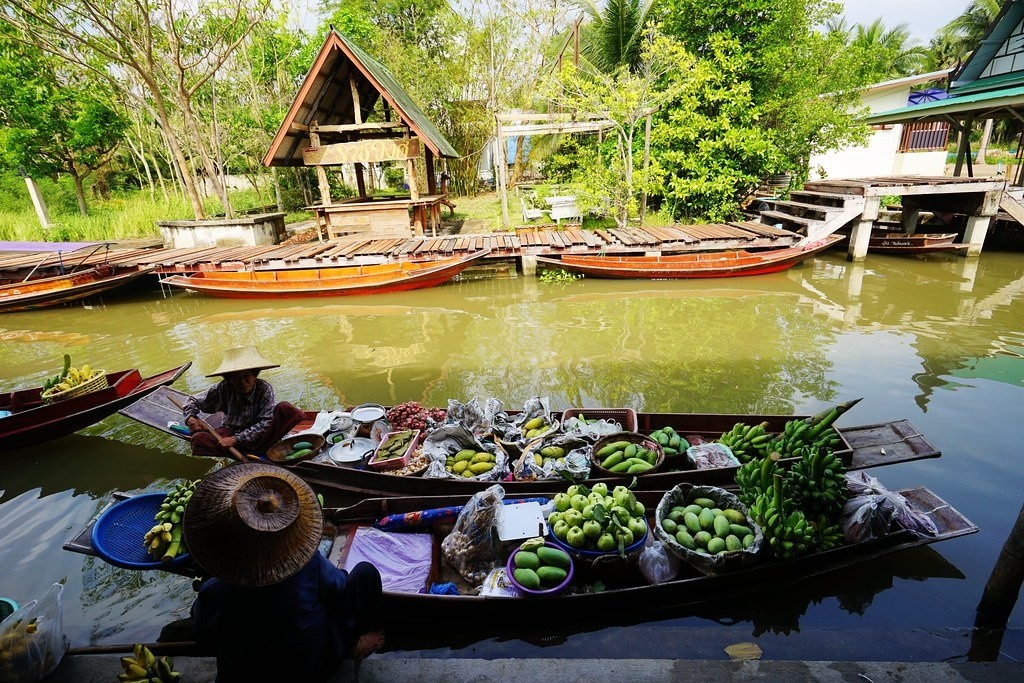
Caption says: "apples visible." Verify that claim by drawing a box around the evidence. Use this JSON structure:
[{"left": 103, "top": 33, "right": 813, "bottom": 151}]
[{"left": 548, "top": 483, "right": 646, "bottom": 549}]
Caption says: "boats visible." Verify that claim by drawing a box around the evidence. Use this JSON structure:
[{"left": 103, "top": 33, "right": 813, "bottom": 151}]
[
  {"left": 834, "top": 228, "right": 979, "bottom": 252},
  {"left": 181, "top": 295, "right": 478, "bottom": 319},
  {"left": 61, "top": 484, "right": 980, "bottom": 608},
  {"left": 554, "top": 276, "right": 809, "bottom": 307},
  {"left": 156, "top": 242, "right": 495, "bottom": 294},
  {"left": 0, "top": 360, "right": 196, "bottom": 447},
  {"left": 118, "top": 383, "right": 946, "bottom": 488},
  {"left": 528, "top": 233, "right": 846, "bottom": 277},
  {"left": 0, "top": 240, "right": 160, "bottom": 314}
]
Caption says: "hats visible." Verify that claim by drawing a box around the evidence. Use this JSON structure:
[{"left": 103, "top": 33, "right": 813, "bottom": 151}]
[
  {"left": 183, "top": 459, "right": 324, "bottom": 587},
  {"left": 205, "top": 344, "right": 281, "bottom": 377}
]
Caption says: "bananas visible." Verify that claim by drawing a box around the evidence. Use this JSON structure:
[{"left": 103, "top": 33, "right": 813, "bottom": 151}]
[
  {"left": 40, "top": 364, "right": 100, "bottom": 396},
  {"left": 720, "top": 418, "right": 849, "bottom": 557},
  {"left": 0, "top": 617, "right": 179, "bottom": 683},
  {"left": 144, "top": 479, "right": 196, "bottom": 559}
]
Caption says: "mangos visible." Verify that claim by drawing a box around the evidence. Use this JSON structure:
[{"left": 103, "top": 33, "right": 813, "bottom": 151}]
[
  {"left": 662, "top": 497, "right": 755, "bottom": 555},
  {"left": 318, "top": 491, "right": 325, "bottom": 509},
  {"left": 284, "top": 442, "right": 313, "bottom": 460},
  {"left": 445, "top": 414, "right": 688, "bottom": 478},
  {"left": 514, "top": 537, "right": 570, "bottom": 588},
  {"left": 334, "top": 434, "right": 345, "bottom": 443}
]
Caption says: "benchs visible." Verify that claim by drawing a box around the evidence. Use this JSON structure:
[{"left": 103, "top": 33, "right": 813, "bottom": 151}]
[
  {"left": 544, "top": 195, "right": 577, "bottom": 207},
  {"left": 520, "top": 198, "right": 544, "bottom": 223},
  {"left": 549, "top": 202, "right": 584, "bottom": 224}
]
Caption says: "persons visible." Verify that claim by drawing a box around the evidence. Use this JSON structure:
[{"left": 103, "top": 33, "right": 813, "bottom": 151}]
[
  {"left": 183, "top": 345, "right": 305, "bottom": 457},
  {"left": 183, "top": 464, "right": 385, "bottom": 683}
]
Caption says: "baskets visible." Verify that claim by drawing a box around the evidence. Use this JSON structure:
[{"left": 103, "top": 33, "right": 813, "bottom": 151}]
[
  {"left": 547, "top": 489, "right": 649, "bottom": 567},
  {"left": 266, "top": 434, "right": 327, "bottom": 466},
  {"left": 654, "top": 482, "right": 764, "bottom": 570},
  {"left": 361, "top": 408, "right": 741, "bottom": 481},
  {"left": 41, "top": 369, "right": 109, "bottom": 404}
]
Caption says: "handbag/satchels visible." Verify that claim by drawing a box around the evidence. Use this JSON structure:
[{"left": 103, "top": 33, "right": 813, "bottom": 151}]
[
  {"left": 0, "top": 583, "right": 68, "bottom": 683},
  {"left": 840, "top": 470, "right": 939, "bottom": 542}
]
